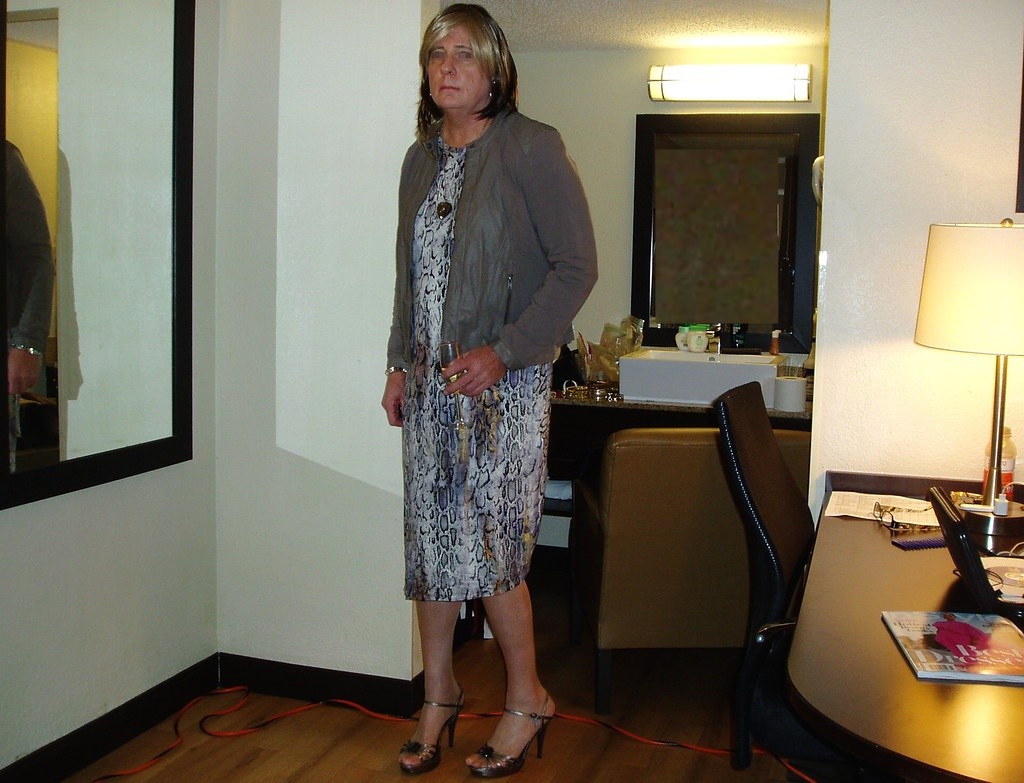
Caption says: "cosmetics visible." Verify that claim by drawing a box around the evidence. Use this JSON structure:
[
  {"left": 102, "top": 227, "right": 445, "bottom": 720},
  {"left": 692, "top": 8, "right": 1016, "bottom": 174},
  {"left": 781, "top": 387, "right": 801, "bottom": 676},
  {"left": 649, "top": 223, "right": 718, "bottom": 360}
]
[
  {"left": 771, "top": 328, "right": 782, "bottom": 354},
  {"left": 676, "top": 322, "right": 759, "bottom": 354}
]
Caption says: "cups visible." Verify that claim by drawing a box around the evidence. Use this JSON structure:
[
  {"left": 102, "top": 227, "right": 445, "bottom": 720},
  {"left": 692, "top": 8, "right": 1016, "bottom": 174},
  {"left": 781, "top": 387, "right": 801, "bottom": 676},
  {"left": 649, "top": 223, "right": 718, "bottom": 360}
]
[{"left": 779, "top": 355, "right": 801, "bottom": 378}]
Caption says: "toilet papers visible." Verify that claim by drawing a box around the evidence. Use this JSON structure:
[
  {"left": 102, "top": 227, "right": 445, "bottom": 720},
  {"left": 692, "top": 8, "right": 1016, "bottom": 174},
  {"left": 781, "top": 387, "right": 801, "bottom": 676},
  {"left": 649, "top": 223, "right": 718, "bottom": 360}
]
[{"left": 774, "top": 376, "right": 807, "bottom": 412}]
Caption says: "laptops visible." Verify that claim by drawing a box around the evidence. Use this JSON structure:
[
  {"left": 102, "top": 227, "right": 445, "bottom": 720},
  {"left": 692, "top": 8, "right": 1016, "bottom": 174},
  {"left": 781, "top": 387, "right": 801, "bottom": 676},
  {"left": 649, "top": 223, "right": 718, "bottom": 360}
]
[{"left": 930, "top": 487, "right": 1024, "bottom": 614}]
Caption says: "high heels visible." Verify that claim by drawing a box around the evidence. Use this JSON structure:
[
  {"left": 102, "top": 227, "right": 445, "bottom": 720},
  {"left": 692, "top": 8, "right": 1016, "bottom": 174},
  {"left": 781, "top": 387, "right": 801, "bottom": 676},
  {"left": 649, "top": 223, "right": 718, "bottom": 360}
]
[
  {"left": 466, "top": 691, "right": 558, "bottom": 779},
  {"left": 398, "top": 683, "right": 465, "bottom": 777}
]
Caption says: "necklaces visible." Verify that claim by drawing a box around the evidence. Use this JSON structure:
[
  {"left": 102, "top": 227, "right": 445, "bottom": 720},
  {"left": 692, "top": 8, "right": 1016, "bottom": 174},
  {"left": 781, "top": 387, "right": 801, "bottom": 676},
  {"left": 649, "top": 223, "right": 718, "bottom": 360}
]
[{"left": 436, "top": 116, "right": 492, "bottom": 220}]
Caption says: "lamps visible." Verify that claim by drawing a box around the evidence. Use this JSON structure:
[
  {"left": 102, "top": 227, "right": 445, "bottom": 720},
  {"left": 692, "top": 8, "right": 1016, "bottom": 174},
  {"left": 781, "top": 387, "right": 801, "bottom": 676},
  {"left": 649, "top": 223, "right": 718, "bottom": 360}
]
[
  {"left": 914, "top": 217, "right": 1024, "bottom": 536},
  {"left": 648, "top": 65, "right": 811, "bottom": 103}
]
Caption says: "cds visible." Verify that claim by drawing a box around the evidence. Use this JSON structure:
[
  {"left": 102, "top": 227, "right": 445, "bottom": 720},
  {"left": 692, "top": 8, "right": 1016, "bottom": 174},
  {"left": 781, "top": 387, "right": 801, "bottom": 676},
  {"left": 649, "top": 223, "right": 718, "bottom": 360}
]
[{"left": 986, "top": 566, "right": 1024, "bottom": 588}]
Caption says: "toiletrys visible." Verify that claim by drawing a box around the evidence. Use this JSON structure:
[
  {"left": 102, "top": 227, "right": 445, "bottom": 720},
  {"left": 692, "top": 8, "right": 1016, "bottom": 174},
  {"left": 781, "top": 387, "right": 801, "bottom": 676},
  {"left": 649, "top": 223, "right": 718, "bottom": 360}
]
[
  {"left": 676, "top": 323, "right": 708, "bottom": 353},
  {"left": 768, "top": 329, "right": 783, "bottom": 355}
]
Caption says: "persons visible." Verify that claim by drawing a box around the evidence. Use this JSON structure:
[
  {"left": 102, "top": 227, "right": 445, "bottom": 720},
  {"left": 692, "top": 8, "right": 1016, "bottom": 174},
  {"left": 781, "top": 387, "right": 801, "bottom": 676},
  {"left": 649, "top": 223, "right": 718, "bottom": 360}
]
[
  {"left": 933, "top": 613, "right": 991, "bottom": 665},
  {"left": 382, "top": 3, "right": 599, "bottom": 779},
  {"left": 0, "top": 137, "right": 54, "bottom": 477}
]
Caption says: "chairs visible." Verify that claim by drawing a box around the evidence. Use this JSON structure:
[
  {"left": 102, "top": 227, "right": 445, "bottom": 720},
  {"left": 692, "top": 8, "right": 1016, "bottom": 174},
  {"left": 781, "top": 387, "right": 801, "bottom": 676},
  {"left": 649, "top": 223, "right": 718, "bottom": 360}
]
[
  {"left": 568, "top": 429, "right": 811, "bottom": 717},
  {"left": 714, "top": 381, "right": 817, "bottom": 769}
]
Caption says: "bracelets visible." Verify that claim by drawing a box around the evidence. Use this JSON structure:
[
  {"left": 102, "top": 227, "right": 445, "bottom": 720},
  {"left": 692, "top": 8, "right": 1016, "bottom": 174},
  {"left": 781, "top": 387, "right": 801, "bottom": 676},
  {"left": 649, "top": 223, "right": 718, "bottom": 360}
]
[
  {"left": 386, "top": 367, "right": 408, "bottom": 376},
  {"left": 11, "top": 341, "right": 41, "bottom": 358}
]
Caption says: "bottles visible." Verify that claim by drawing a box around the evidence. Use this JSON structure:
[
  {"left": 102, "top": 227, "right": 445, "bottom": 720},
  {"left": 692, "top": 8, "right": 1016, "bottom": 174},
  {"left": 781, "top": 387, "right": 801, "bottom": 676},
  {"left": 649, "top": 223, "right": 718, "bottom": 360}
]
[
  {"left": 983, "top": 426, "right": 1017, "bottom": 502},
  {"left": 803, "top": 336, "right": 816, "bottom": 402}
]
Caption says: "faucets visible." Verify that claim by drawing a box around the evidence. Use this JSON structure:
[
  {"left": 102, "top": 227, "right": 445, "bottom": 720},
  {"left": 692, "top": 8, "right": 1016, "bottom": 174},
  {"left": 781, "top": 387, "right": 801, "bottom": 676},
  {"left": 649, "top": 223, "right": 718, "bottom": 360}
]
[{"left": 703, "top": 325, "right": 725, "bottom": 354}]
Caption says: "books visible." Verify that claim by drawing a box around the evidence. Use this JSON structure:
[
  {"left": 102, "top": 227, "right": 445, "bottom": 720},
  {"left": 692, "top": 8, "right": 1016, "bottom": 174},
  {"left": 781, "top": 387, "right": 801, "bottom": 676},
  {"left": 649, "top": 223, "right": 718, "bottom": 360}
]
[{"left": 882, "top": 610, "right": 1023, "bottom": 682}]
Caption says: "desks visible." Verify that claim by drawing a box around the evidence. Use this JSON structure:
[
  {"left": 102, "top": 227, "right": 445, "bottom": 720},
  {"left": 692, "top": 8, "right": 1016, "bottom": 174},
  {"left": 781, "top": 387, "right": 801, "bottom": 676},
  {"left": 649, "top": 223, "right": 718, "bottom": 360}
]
[{"left": 788, "top": 491, "right": 1024, "bottom": 783}]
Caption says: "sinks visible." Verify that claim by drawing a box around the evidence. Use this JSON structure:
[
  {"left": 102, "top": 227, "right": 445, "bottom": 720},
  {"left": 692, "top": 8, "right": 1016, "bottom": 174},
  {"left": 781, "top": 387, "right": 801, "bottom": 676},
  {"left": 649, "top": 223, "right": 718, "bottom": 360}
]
[{"left": 618, "top": 347, "right": 789, "bottom": 410}]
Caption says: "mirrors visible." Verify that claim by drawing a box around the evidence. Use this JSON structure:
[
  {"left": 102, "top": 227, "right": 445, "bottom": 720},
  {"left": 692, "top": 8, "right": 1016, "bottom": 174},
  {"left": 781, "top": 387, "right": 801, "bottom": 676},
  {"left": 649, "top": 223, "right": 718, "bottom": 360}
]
[
  {"left": 0, "top": 0, "right": 195, "bottom": 510},
  {"left": 630, "top": 113, "right": 800, "bottom": 354}
]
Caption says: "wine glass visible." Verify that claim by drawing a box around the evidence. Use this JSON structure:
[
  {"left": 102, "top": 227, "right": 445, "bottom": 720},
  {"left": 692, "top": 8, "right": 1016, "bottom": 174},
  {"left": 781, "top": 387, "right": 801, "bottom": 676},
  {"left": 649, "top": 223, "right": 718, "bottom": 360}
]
[{"left": 439, "top": 340, "right": 473, "bottom": 430}]
